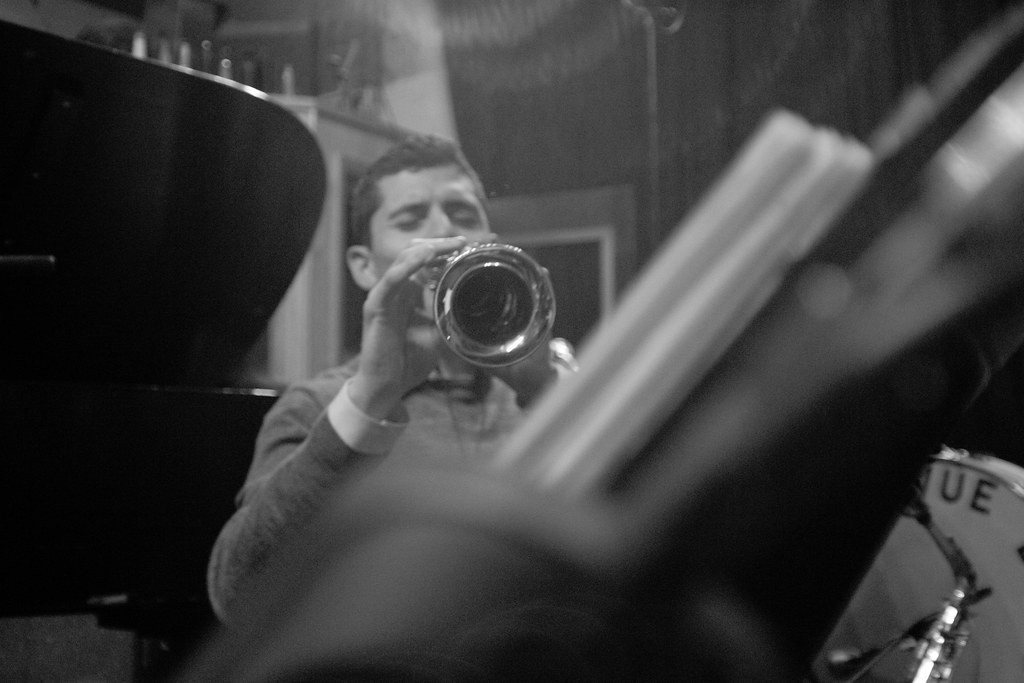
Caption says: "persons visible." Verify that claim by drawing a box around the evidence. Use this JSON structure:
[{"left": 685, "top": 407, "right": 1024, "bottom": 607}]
[{"left": 207, "top": 133, "right": 576, "bottom": 631}]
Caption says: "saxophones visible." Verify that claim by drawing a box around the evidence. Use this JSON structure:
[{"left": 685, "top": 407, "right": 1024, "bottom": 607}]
[{"left": 903, "top": 495, "right": 981, "bottom": 681}]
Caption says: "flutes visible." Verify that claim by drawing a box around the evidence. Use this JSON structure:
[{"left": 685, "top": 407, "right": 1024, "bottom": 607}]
[{"left": 428, "top": 240, "right": 557, "bottom": 369}]
